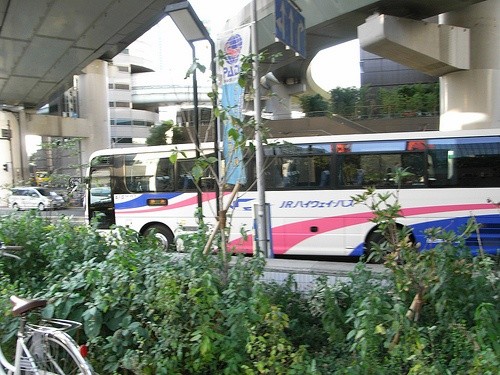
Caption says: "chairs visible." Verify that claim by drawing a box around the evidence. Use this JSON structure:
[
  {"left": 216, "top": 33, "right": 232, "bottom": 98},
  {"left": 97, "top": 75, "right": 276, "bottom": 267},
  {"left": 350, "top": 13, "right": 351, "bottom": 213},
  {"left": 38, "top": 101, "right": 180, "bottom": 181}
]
[
  {"left": 319, "top": 170, "right": 332, "bottom": 187},
  {"left": 352, "top": 168, "right": 365, "bottom": 186},
  {"left": 183, "top": 168, "right": 202, "bottom": 190}
]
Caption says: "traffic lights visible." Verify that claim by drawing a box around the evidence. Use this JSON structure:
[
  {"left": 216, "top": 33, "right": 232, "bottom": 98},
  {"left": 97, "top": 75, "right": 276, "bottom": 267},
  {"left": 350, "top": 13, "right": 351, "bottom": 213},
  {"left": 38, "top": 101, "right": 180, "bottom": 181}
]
[{"left": 3, "top": 163, "right": 8, "bottom": 172}]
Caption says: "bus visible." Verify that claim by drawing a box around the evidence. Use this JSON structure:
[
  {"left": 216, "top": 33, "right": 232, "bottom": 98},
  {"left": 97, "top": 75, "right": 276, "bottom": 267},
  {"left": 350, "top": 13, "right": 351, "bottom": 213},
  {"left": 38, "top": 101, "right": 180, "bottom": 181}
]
[{"left": 68, "top": 129, "right": 500, "bottom": 268}]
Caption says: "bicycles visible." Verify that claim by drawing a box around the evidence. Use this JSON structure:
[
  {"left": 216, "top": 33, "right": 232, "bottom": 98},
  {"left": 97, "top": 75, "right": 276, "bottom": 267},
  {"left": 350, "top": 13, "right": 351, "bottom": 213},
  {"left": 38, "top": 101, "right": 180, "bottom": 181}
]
[{"left": 0, "top": 243, "right": 97, "bottom": 375}]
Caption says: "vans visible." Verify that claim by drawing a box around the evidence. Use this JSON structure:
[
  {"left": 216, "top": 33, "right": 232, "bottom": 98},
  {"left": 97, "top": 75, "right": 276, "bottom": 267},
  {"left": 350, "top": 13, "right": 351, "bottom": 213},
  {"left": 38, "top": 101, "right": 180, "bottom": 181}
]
[{"left": 9, "top": 187, "right": 65, "bottom": 211}]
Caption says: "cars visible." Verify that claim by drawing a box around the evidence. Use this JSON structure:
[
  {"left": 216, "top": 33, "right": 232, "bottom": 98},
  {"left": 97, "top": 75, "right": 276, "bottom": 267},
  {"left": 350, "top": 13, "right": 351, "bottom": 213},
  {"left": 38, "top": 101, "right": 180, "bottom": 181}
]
[{"left": 25, "top": 171, "right": 51, "bottom": 186}]
